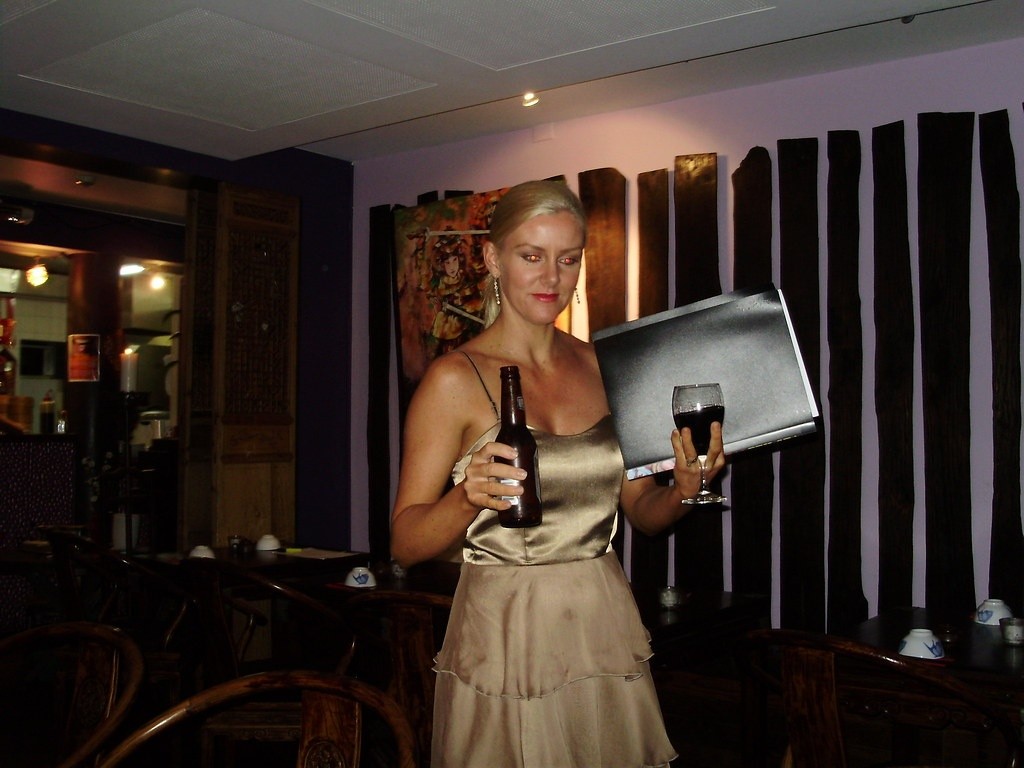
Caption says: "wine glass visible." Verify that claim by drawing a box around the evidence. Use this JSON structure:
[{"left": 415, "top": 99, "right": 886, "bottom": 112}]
[{"left": 672, "top": 385, "right": 730, "bottom": 503}]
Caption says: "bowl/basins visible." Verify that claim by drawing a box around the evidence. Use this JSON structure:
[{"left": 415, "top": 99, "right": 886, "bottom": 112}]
[
  {"left": 256, "top": 534, "right": 282, "bottom": 551},
  {"left": 191, "top": 546, "right": 216, "bottom": 558},
  {"left": 899, "top": 628, "right": 945, "bottom": 659},
  {"left": 977, "top": 599, "right": 1013, "bottom": 625},
  {"left": 346, "top": 566, "right": 377, "bottom": 587}
]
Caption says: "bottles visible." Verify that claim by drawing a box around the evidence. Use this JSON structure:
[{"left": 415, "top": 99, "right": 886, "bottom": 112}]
[
  {"left": 41, "top": 397, "right": 56, "bottom": 433},
  {"left": 494, "top": 366, "right": 544, "bottom": 528}
]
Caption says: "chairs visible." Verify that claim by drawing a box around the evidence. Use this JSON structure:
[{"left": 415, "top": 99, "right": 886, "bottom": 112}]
[
  {"left": 36, "top": 523, "right": 199, "bottom": 652},
  {"left": 95, "top": 670, "right": 420, "bottom": 768},
  {"left": 650, "top": 630, "right": 1023, "bottom": 768},
  {"left": 345, "top": 590, "right": 455, "bottom": 752},
  {"left": 0, "top": 621, "right": 143, "bottom": 768},
  {"left": 179, "top": 557, "right": 358, "bottom": 688}
]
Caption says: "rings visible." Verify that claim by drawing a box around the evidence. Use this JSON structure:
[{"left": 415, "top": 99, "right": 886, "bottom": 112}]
[{"left": 686, "top": 456, "right": 698, "bottom": 466}]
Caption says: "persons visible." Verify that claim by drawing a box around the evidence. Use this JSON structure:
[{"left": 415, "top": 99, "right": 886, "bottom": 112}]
[{"left": 388, "top": 181, "right": 727, "bottom": 768}]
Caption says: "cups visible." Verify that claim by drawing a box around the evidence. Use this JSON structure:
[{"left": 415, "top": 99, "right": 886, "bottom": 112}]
[{"left": 999, "top": 617, "right": 1024, "bottom": 645}]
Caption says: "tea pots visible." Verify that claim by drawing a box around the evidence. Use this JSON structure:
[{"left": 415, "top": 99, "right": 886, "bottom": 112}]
[{"left": 231, "top": 538, "right": 256, "bottom": 555}]
